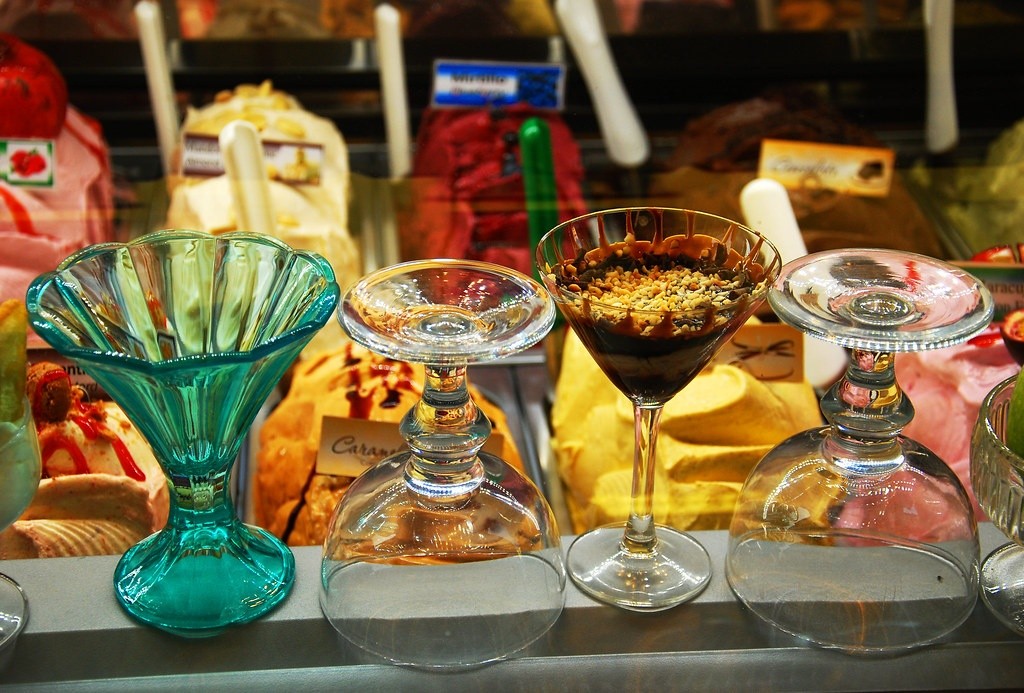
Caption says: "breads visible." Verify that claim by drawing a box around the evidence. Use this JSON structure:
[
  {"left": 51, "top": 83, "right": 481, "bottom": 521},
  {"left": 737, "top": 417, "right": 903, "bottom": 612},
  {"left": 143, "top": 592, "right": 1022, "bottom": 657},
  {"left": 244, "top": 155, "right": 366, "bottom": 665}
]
[{"left": 613, "top": 363, "right": 797, "bottom": 484}]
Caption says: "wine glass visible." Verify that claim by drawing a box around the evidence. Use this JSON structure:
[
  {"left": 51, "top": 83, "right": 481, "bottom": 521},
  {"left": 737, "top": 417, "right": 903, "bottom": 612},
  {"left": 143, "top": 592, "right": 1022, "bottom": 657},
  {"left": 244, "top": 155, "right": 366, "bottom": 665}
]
[
  {"left": 0, "top": 393, "right": 42, "bottom": 646},
  {"left": 535, "top": 207, "right": 782, "bottom": 613},
  {"left": 24, "top": 229, "right": 340, "bottom": 638},
  {"left": 725, "top": 247, "right": 994, "bottom": 656},
  {"left": 969, "top": 373, "right": 1024, "bottom": 636},
  {"left": 317, "top": 259, "right": 567, "bottom": 671}
]
